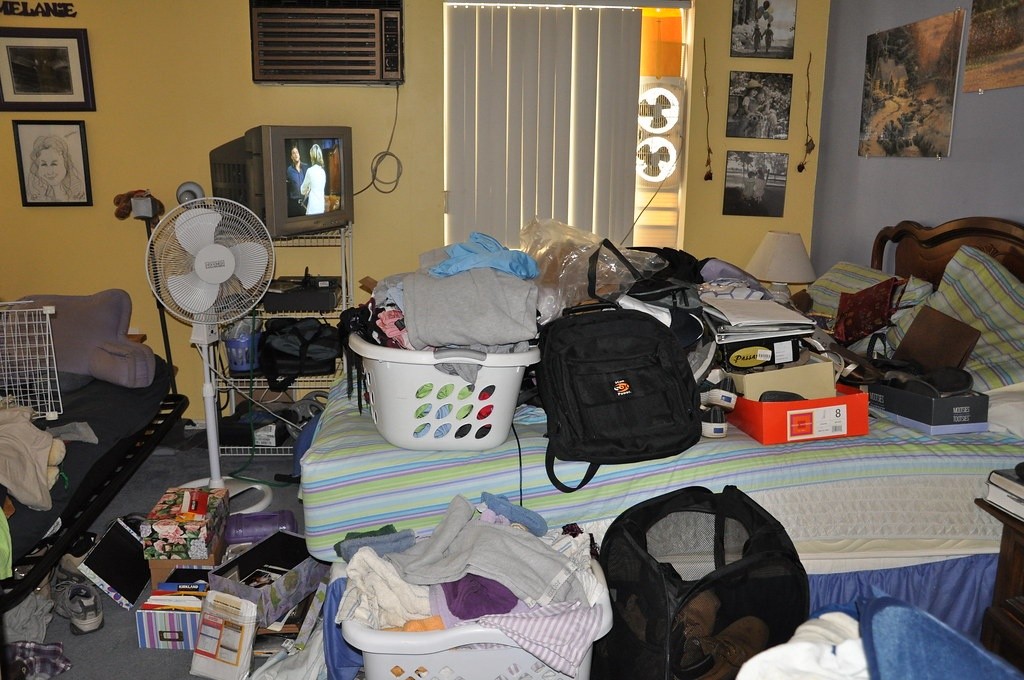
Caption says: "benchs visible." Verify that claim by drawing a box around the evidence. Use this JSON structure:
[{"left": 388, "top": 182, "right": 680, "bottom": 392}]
[{"left": 0, "top": 332, "right": 190, "bottom": 680}]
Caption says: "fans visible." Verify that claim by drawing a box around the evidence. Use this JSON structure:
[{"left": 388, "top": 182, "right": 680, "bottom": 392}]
[
  {"left": 145, "top": 198, "right": 275, "bottom": 517},
  {"left": 638, "top": 76, "right": 686, "bottom": 193}
]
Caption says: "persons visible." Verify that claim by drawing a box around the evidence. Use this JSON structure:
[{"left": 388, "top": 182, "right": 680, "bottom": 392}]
[
  {"left": 287, "top": 145, "right": 308, "bottom": 218},
  {"left": 300, "top": 144, "right": 327, "bottom": 215},
  {"left": 742, "top": 79, "right": 770, "bottom": 138},
  {"left": 752, "top": 24, "right": 762, "bottom": 53},
  {"left": 743, "top": 170, "right": 766, "bottom": 207},
  {"left": 761, "top": 23, "right": 774, "bottom": 52}
]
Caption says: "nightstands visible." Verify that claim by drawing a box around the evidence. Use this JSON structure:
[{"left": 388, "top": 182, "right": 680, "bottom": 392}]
[{"left": 974, "top": 498, "right": 1024, "bottom": 673}]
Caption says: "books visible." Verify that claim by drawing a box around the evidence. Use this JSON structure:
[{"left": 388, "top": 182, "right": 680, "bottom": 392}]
[
  {"left": 983, "top": 469, "right": 1024, "bottom": 522},
  {"left": 141, "top": 582, "right": 209, "bottom": 611}
]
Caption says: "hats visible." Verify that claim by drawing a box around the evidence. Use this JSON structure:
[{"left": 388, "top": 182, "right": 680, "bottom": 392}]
[{"left": 747, "top": 80, "right": 761, "bottom": 89}]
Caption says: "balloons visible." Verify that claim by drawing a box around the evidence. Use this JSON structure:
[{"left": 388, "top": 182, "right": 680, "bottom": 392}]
[{"left": 756, "top": 1, "right": 774, "bottom": 22}]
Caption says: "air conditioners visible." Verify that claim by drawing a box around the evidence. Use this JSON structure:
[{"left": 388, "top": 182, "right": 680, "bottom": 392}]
[{"left": 250, "top": 6, "right": 402, "bottom": 82}]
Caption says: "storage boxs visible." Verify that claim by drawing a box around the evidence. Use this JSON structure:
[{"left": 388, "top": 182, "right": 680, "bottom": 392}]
[
  {"left": 724, "top": 333, "right": 990, "bottom": 446},
  {"left": 140, "top": 487, "right": 332, "bottom": 628},
  {"left": 264, "top": 275, "right": 341, "bottom": 311}
]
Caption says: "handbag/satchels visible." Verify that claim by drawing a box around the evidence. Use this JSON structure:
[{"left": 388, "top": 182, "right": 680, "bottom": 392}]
[
  {"left": 590, "top": 484, "right": 809, "bottom": 680},
  {"left": 836, "top": 276, "right": 909, "bottom": 342},
  {"left": 541, "top": 302, "right": 704, "bottom": 465}
]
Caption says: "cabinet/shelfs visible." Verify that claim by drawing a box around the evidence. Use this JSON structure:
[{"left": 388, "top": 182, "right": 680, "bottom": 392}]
[{"left": 209, "top": 220, "right": 355, "bottom": 472}]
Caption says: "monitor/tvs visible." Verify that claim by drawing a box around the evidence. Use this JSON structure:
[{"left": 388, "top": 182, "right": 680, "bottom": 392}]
[{"left": 209, "top": 125, "right": 354, "bottom": 238}]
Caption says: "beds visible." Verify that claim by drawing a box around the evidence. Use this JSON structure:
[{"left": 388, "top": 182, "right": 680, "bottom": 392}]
[{"left": 298, "top": 215, "right": 1024, "bottom": 680}]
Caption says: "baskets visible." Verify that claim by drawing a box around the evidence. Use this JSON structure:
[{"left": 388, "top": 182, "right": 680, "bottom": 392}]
[
  {"left": 342, "top": 558, "right": 614, "bottom": 680},
  {"left": 221, "top": 331, "right": 262, "bottom": 372},
  {"left": 348, "top": 332, "right": 540, "bottom": 452}
]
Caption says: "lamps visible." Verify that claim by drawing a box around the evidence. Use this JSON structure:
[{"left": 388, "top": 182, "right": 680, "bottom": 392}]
[{"left": 743, "top": 230, "right": 817, "bottom": 304}]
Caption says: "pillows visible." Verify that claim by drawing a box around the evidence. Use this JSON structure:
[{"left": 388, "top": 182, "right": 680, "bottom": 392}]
[
  {"left": 885, "top": 245, "right": 1024, "bottom": 392},
  {"left": 805, "top": 260, "right": 932, "bottom": 356}
]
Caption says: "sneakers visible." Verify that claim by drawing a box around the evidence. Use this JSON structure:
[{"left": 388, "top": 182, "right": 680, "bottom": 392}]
[
  {"left": 698, "top": 368, "right": 738, "bottom": 439},
  {"left": 672, "top": 613, "right": 771, "bottom": 680},
  {"left": 47, "top": 531, "right": 106, "bottom": 634}
]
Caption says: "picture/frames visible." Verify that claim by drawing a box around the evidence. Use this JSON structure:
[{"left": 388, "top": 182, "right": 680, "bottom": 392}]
[
  {"left": 0, "top": 27, "right": 97, "bottom": 113},
  {"left": 12, "top": 119, "right": 93, "bottom": 207}
]
